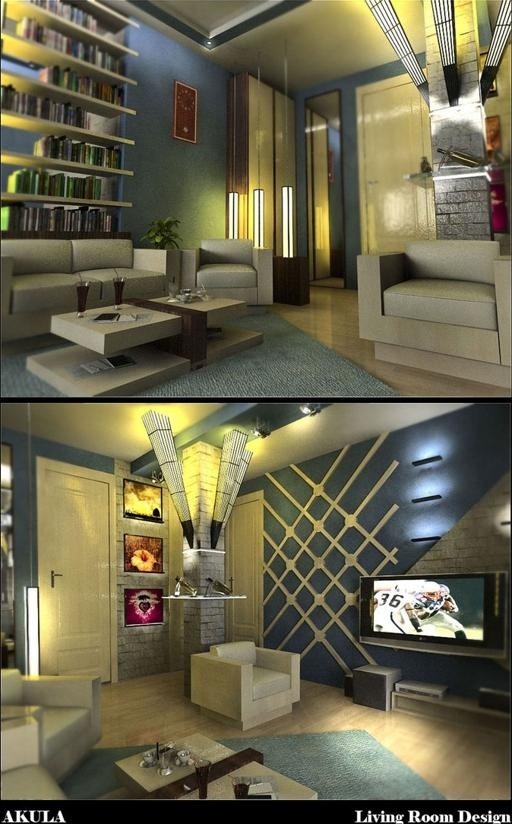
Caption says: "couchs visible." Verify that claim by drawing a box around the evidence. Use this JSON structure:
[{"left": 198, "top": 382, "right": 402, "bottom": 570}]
[{"left": 1, "top": 240, "right": 180, "bottom": 341}]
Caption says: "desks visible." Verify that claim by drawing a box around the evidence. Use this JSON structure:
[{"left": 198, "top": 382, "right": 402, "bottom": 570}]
[{"left": 116, "top": 733, "right": 318, "bottom": 800}]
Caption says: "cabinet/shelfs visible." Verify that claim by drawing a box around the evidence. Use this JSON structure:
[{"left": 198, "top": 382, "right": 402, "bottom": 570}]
[
  {"left": 1, "top": 0, "right": 139, "bottom": 236},
  {"left": 392, "top": 691, "right": 512, "bottom": 737}
]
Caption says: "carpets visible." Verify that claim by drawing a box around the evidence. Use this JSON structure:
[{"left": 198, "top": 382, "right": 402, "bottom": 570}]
[
  {"left": 61, "top": 729, "right": 446, "bottom": 799},
  {"left": 1, "top": 306, "right": 399, "bottom": 398}
]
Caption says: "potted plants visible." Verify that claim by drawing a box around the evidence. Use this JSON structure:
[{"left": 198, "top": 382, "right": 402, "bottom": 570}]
[{"left": 138, "top": 217, "right": 183, "bottom": 294}]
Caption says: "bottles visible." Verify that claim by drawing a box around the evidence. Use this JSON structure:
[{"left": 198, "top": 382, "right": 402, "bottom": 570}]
[
  {"left": 174, "top": 575, "right": 198, "bottom": 597},
  {"left": 208, "top": 577, "right": 231, "bottom": 596}
]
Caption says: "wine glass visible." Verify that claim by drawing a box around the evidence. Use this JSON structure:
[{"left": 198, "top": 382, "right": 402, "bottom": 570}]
[{"left": 168, "top": 280, "right": 179, "bottom": 303}]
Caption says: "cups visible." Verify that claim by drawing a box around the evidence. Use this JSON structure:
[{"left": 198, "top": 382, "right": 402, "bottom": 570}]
[
  {"left": 113, "top": 278, "right": 125, "bottom": 310},
  {"left": 232, "top": 777, "right": 251, "bottom": 799},
  {"left": 144, "top": 753, "right": 154, "bottom": 767},
  {"left": 76, "top": 282, "right": 90, "bottom": 317},
  {"left": 177, "top": 751, "right": 190, "bottom": 767}
]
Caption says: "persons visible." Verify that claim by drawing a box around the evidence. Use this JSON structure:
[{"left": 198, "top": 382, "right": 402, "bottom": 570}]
[{"left": 366, "top": 578, "right": 469, "bottom": 641}]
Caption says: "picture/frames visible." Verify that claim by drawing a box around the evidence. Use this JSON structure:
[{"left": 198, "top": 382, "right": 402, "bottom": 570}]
[
  {"left": 125, "top": 534, "right": 165, "bottom": 574},
  {"left": 123, "top": 478, "right": 164, "bottom": 523},
  {"left": 172, "top": 79, "right": 198, "bottom": 143},
  {"left": 124, "top": 588, "right": 166, "bottom": 628},
  {"left": 479, "top": 51, "right": 499, "bottom": 98}
]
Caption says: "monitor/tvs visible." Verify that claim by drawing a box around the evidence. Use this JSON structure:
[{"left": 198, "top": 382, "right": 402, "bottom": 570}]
[{"left": 359, "top": 570, "right": 512, "bottom": 660}]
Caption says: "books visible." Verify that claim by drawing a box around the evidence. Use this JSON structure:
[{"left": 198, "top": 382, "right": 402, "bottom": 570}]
[
  {"left": 94, "top": 310, "right": 138, "bottom": 324},
  {"left": 1, "top": 1, "right": 129, "bottom": 236},
  {"left": 79, "top": 353, "right": 138, "bottom": 375}
]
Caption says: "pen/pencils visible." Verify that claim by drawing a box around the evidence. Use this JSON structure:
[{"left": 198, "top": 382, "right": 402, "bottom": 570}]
[{"left": 116, "top": 315, "right": 120, "bottom": 321}]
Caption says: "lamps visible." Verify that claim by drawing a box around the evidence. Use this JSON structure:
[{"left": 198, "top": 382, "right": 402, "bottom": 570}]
[
  {"left": 298, "top": 403, "right": 322, "bottom": 417},
  {"left": 252, "top": 66, "right": 264, "bottom": 249},
  {"left": 25, "top": 404, "right": 41, "bottom": 677},
  {"left": 229, "top": 73, "right": 238, "bottom": 238},
  {"left": 282, "top": 38, "right": 296, "bottom": 258}
]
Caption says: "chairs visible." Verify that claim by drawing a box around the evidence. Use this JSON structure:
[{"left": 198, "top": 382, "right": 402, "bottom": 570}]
[
  {"left": 181, "top": 237, "right": 274, "bottom": 306},
  {"left": 0, "top": 717, "right": 66, "bottom": 799},
  {"left": 0, "top": 668, "right": 101, "bottom": 784},
  {"left": 356, "top": 239, "right": 512, "bottom": 388},
  {"left": 191, "top": 641, "right": 301, "bottom": 730}
]
